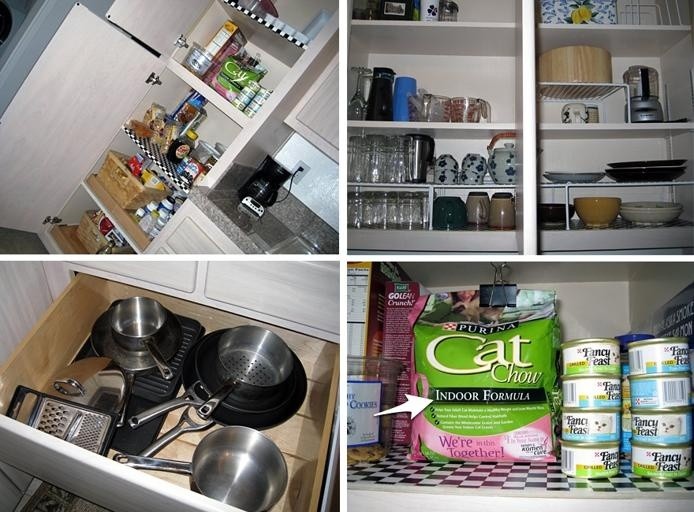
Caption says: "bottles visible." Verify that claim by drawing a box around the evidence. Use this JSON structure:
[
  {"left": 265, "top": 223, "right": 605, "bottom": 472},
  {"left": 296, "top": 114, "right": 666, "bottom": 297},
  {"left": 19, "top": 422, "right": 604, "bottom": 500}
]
[
  {"left": 95, "top": 240, "right": 115, "bottom": 254},
  {"left": 420, "top": 0, "right": 459, "bottom": 21},
  {"left": 133, "top": 192, "right": 188, "bottom": 238}
]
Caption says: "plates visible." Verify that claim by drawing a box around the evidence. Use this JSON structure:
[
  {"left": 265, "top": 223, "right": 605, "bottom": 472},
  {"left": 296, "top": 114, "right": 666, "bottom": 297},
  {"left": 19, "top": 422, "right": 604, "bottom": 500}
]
[{"left": 542, "top": 158, "right": 688, "bottom": 184}]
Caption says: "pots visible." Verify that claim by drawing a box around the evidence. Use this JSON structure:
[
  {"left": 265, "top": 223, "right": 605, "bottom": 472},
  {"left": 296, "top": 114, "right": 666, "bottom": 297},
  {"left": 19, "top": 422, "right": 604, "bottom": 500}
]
[
  {"left": 126, "top": 325, "right": 308, "bottom": 458},
  {"left": 112, "top": 425, "right": 288, "bottom": 511},
  {"left": 90, "top": 297, "right": 183, "bottom": 428}
]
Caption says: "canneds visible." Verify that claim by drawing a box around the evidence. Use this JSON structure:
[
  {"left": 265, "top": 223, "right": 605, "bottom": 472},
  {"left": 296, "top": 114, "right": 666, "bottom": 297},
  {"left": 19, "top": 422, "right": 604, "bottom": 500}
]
[
  {"left": 559, "top": 336, "right": 694, "bottom": 480},
  {"left": 232, "top": 80, "right": 271, "bottom": 119}
]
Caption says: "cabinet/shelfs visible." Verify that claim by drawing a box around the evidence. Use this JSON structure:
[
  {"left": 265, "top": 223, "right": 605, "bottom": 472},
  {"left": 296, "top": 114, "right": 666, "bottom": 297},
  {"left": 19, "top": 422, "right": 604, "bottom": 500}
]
[
  {"left": 0, "top": 261, "right": 339, "bottom": 512},
  {"left": 0, "top": 0, "right": 339, "bottom": 254},
  {"left": 347, "top": 0, "right": 694, "bottom": 256}
]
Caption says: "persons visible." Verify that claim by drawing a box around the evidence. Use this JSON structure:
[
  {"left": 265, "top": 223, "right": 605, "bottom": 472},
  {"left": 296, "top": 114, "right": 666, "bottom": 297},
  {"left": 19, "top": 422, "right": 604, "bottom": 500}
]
[{"left": 443, "top": 290, "right": 479, "bottom": 311}]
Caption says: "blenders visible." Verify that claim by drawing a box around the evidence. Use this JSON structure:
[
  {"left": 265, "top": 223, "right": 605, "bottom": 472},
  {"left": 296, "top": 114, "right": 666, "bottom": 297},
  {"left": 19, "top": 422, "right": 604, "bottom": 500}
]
[{"left": 623, "top": 64, "right": 664, "bottom": 122}]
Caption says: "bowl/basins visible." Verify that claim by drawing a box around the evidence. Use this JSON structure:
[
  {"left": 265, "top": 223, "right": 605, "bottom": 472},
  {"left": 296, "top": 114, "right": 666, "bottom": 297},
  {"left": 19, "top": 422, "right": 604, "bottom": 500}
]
[
  {"left": 618, "top": 201, "right": 685, "bottom": 227},
  {"left": 537, "top": 45, "right": 612, "bottom": 98},
  {"left": 538, "top": 197, "right": 621, "bottom": 228}
]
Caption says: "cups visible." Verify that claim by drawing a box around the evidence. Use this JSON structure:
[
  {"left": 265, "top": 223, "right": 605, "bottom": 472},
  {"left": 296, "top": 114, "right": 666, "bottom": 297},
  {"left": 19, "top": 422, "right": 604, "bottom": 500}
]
[
  {"left": 348, "top": 133, "right": 488, "bottom": 185},
  {"left": 464, "top": 192, "right": 516, "bottom": 230},
  {"left": 347, "top": 355, "right": 407, "bottom": 468},
  {"left": 432, "top": 196, "right": 468, "bottom": 230},
  {"left": 365, "top": 66, "right": 488, "bottom": 123},
  {"left": 348, "top": 190, "right": 430, "bottom": 231}
]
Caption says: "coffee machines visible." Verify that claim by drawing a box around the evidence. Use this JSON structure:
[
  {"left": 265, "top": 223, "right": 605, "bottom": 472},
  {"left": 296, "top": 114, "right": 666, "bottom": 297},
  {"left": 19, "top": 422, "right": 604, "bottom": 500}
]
[{"left": 236, "top": 154, "right": 294, "bottom": 220}]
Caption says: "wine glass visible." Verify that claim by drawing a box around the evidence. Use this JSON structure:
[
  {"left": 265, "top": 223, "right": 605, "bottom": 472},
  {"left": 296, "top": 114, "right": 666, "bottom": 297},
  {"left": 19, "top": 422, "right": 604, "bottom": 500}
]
[{"left": 347, "top": 65, "right": 374, "bottom": 121}]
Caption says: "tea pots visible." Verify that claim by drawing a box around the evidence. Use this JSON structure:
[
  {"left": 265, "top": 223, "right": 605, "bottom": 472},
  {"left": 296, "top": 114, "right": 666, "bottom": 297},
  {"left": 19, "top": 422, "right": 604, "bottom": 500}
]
[{"left": 487, "top": 133, "right": 543, "bottom": 185}]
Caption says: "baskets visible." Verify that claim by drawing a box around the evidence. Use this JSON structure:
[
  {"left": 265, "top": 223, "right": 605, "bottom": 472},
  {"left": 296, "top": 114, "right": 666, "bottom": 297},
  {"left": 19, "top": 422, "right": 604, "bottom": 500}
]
[
  {"left": 74, "top": 207, "right": 133, "bottom": 253},
  {"left": 94, "top": 147, "right": 175, "bottom": 212}
]
